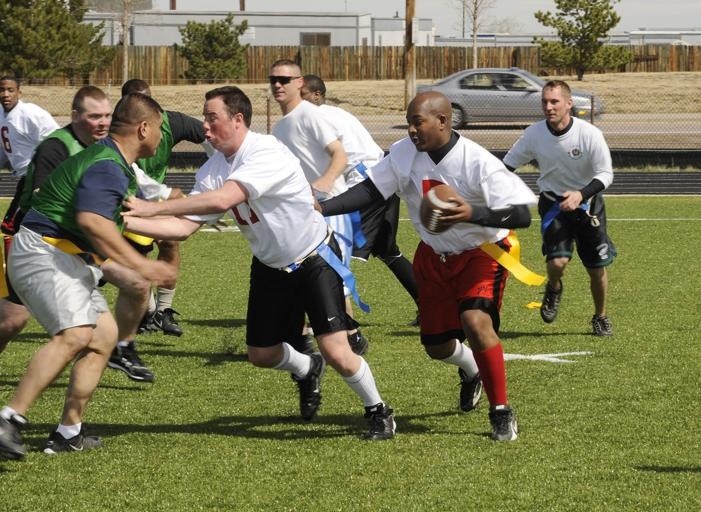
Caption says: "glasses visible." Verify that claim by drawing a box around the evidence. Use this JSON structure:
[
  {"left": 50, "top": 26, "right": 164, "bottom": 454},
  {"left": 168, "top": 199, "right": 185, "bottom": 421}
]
[{"left": 268, "top": 75, "right": 298, "bottom": 85}]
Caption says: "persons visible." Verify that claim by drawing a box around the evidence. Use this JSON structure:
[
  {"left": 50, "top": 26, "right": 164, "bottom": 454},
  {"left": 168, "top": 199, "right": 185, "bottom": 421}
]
[
  {"left": 0, "top": 75, "right": 63, "bottom": 181},
  {"left": 121, "top": 78, "right": 229, "bottom": 337},
  {"left": 119, "top": 85, "right": 397, "bottom": 441},
  {"left": 314, "top": 90, "right": 540, "bottom": 442},
  {"left": 1, "top": 86, "right": 184, "bottom": 384},
  {"left": 267, "top": 59, "right": 369, "bottom": 358},
  {"left": 1, "top": 91, "right": 180, "bottom": 463},
  {"left": 302, "top": 74, "right": 421, "bottom": 327},
  {"left": 502, "top": 79, "right": 617, "bottom": 336}
]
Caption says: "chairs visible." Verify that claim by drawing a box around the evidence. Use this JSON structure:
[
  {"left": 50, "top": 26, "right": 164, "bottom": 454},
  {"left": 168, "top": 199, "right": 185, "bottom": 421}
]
[{"left": 491, "top": 76, "right": 508, "bottom": 91}]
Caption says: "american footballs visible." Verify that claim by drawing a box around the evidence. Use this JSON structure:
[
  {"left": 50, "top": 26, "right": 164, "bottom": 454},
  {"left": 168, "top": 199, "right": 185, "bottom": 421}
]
[{"left": 420, "top": 185, "right": 463, "bottom": 236}]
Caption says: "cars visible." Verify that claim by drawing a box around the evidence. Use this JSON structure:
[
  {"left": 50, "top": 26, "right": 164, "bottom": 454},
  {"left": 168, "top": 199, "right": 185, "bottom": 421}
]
[{"left": 416, "top": 66, "right": 603, "bottom": 134}]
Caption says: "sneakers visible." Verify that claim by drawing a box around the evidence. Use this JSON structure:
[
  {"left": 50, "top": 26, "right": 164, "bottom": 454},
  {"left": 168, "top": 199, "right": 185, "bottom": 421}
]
[
  {"left": 292, "top": 333, "right": 324, "bottom": 360},
  {"left": 0, "top": 410, "right": 29, "bottom": 461},
  {"left": 45, "top": 426, "right": 104, "bottom": 454},
  {"left": 348, "top": 327, "right": 369, "bottom": 355},
  {"left": 138, "top": 308, "right": 161, "bottom": 335},
  {"left": 291, "top": 351, "right": 326, "bottom": 421},
  {"left": 457, "top": 367, "right": 483, "bottom": 411},
  {"left": 590, "top": 315, "right": 611, "bottom": 337},
  {"left": 487, "top": 403, "right": 518, "bottom": 441},
  {"left": 108, "top": 340, "right": 154, "bottom": 383},
  {"left": 539, "top": 280, "right": 563, "bottom": 323},
  {"left": 406, "top": 310, "right": 421, "bottom": 327},
  {"left": 154, "top": 307, "right": 183, "bottom": 337},
  {"left": 363, "top": 403, "right": 397, "bottom": 441}
]
